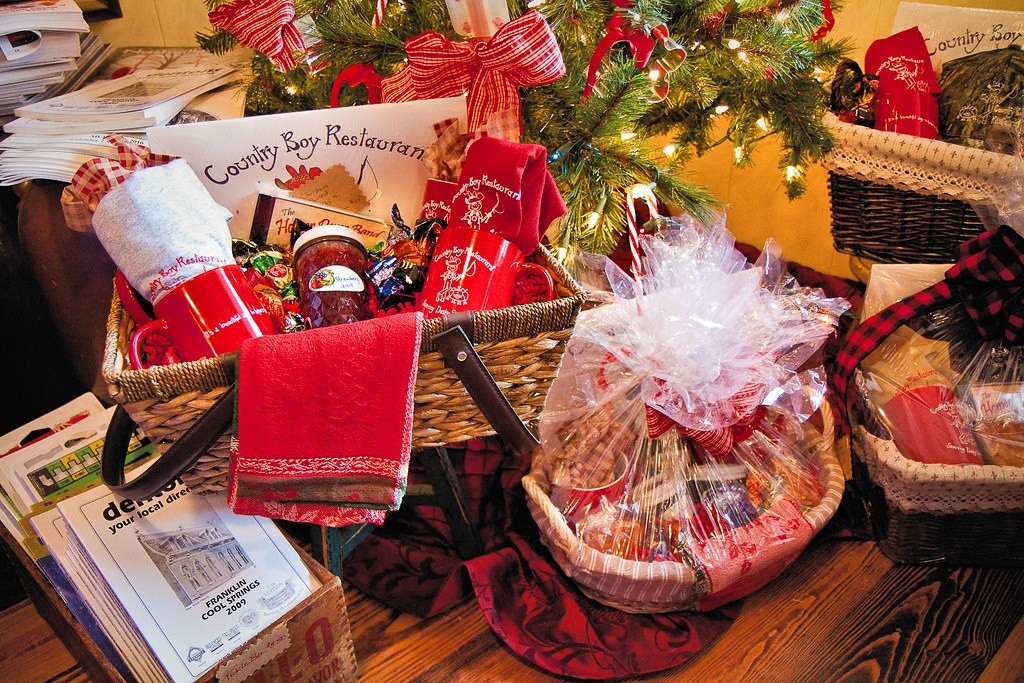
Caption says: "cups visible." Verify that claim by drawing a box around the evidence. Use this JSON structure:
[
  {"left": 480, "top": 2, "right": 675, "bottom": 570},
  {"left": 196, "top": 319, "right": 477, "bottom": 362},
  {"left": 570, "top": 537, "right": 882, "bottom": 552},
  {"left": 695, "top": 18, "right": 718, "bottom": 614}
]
[
  {"left": 420, "top": 228, "right": 554, "bottom": 319},
  {"left": 128, "top": 264, "right": 279, "bottom": 370},
  {"left": 635, "top": 474, "right": 688, "bottom": 521},
  {"left": 691, "top": 464, "right": 746, "bottom": 504},
  {"left": 883, "top": 385, "right": 983, "bottom": 466},
  {"left": 875, "top": 89, "right": 940, "bottom": 140},
  {"left": 551, "top": 450, "right": 628, "bottom": 534}
]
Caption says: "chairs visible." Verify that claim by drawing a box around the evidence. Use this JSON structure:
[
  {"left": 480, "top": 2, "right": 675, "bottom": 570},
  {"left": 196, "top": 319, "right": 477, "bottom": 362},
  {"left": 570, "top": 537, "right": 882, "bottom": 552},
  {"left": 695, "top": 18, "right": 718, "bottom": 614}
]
[{"left": 17, "top": 109, "right": 216, "bottom": 408}]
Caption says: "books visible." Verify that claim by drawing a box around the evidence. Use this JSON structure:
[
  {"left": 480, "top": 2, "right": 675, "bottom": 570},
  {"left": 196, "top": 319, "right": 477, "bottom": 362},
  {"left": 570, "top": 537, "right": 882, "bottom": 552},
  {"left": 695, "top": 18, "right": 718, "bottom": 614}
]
[{"left": 0, "top": 0, "right": 244, "bottom": 187}]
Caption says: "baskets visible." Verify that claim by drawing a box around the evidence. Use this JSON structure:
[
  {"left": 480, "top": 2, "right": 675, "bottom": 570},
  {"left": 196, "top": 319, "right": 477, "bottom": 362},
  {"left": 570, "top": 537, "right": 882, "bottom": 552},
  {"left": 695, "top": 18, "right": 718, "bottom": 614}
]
[
  {"left": 100, "top": 245, "right": 582, "bottom": 497},
  {"left": 820, "top": 106, "right": 1024, "bottom": 263},
  {"left": 522, "top": 348, "right": 843, "bottom": 614},
  {"left": 847, "top": 363, "right": 1024, "bottom": 515}
]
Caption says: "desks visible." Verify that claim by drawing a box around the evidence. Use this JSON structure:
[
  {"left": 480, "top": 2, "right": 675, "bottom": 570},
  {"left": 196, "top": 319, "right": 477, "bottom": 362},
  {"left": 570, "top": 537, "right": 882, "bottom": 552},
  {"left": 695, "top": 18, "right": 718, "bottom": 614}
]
[{"left": 0, "top": 241, "right": 1024, "bottom": 683}]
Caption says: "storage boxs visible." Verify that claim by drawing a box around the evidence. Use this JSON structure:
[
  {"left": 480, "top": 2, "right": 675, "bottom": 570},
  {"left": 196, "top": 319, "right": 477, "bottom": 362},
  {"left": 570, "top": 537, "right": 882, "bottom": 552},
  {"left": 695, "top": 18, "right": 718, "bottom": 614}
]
[{"left": 0, "top": 520, "right": 360, "bottom": 683}]
